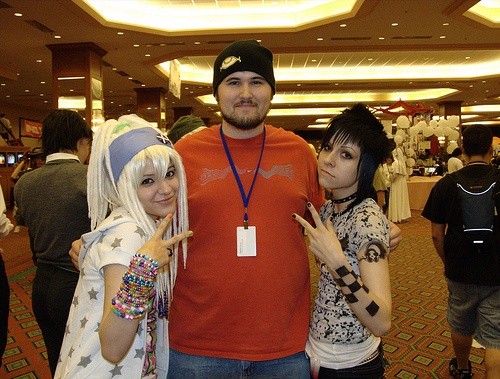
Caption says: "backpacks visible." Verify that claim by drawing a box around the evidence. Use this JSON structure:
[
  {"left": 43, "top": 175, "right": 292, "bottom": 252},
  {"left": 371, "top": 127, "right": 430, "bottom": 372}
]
[{"left": 444, "top": 172, "right": 498, "bottom": 273}]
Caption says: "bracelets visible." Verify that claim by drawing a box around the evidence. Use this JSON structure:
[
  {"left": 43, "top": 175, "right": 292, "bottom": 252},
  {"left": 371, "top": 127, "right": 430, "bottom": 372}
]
[{"left": 109, "top": 254, "right": 160, "bottom": 319}]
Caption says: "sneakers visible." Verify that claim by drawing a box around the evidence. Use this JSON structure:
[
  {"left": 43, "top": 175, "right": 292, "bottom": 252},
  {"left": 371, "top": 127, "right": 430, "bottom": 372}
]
[{"left": 449, "top": 358, "right": 473, "bottom": 378}]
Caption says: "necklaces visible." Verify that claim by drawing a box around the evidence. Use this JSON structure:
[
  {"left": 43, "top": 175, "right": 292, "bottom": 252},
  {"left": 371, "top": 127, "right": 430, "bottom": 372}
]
[{"left": 330, "top": 191, "right": 357, "bottom": 204}]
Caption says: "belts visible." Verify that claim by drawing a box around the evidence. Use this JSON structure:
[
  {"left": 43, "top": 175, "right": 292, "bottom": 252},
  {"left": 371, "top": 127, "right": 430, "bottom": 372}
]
[{"left": 358, "top": 347, "right": 380, "bottom": 366}]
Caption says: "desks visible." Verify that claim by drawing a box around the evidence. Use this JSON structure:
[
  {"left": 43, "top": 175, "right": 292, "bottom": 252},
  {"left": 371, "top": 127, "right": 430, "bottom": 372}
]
[{"left": 406, "top": 175, "right": 443, "bottom": 210}]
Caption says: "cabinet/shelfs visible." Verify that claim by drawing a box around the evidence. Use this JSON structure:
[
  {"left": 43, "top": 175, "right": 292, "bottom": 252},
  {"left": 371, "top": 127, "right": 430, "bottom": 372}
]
[{"left": 0, "top": 145, "right": 32, "bottom": 210}]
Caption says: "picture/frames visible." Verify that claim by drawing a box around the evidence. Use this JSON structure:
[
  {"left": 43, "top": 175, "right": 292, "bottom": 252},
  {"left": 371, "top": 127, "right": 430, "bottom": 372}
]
[{"left": 19, "top": 117, "right": 43, "bottom": 139}]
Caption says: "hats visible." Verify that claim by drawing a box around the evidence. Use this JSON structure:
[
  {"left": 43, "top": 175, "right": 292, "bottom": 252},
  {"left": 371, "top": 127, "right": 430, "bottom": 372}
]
[
  {"left": 168, "top": 115, "right": 205, "bottom": 142},
  {"left": 212, "top": 39, "right": 277, "bottom": 96}
]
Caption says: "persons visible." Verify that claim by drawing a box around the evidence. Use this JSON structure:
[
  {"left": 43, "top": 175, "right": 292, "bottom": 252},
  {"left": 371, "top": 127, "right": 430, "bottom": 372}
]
[
  {"left": 291, "top": 105, "right": 392, "bottom": 379},
  {"left": 0, "top": 113, "right": 16, "bottom": 144},
  {"left": 52, "top": 113, "right": 193, "bottom": 379},
  {"left": 1, "top": 187, "right": 14, "bottom": 363},
  {"left": 432, "top": 161, "right": 444, "bottom": 176},
  {"left": 12, "top": 157, "right": 32, "bottom": 234},
  {"left": 69, "top": 39, "right": 400, "bottom": 379},
  {"left": 421, "top": 124, "right": 500, "bottom": 379},
  {"left": 10, "top": 147, "right": 46, "bottom": 184},
  {"left": 15, "top": 110, "right": 110, "bottom": 378},
  {"left": 447, "top": 148, "right": 464, "bottom": 174},
  {"left": 388, "top": 148, "right": 411, "bottom": 223},
  {"left": 380, "top": 156, "right": 394, "bottom": 215}
]
